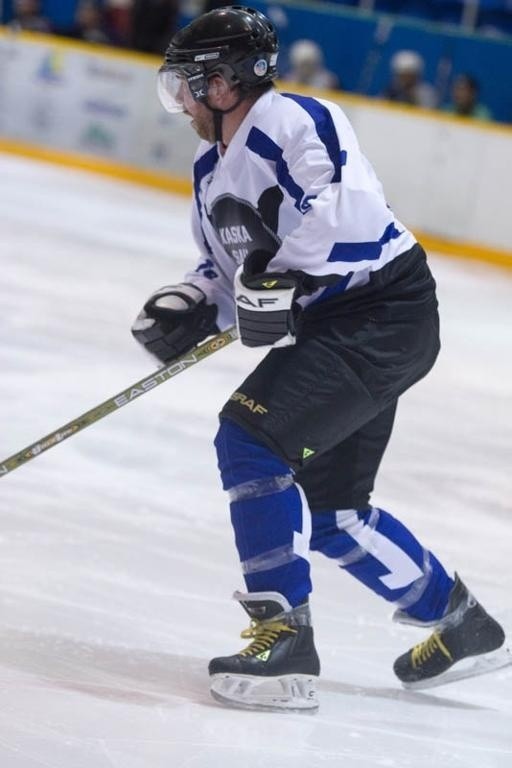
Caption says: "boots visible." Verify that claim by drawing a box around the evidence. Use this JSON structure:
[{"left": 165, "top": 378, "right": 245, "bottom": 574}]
[
  {"left": 209, "top": 591, "right": 319, "bottom": 677},
  {"left": 392, "top": 571, "right": 505, "bottom": 682}
]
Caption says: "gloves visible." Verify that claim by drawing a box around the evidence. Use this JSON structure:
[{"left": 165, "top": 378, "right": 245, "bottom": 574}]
[
  {"left": 131, "top": 282, "right": 221, "bottom": 363},
  {"left": 234, "top": 250, "right": 312, "bottom": 348}
]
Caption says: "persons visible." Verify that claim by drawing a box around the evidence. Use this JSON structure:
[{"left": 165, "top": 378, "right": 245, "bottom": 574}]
[
  {"left": 441, "top": 73, "right": 493, "bottom": 121},
  {"left": 4, "top": 1, "right": 184, "bottom": 56},
  {"left": 283, "top": 38, "right": 340, "bottom": 91},
  {"left": 381, "top": 50, "right": 438, "bottom": 110},
  {"left": 126, "top": 3, "right": 507, "bottom": 684}
]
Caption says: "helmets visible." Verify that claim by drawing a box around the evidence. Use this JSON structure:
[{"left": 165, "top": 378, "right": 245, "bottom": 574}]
[{"left": 157, "top": 5, "right": 280, "bottom": 114}]
